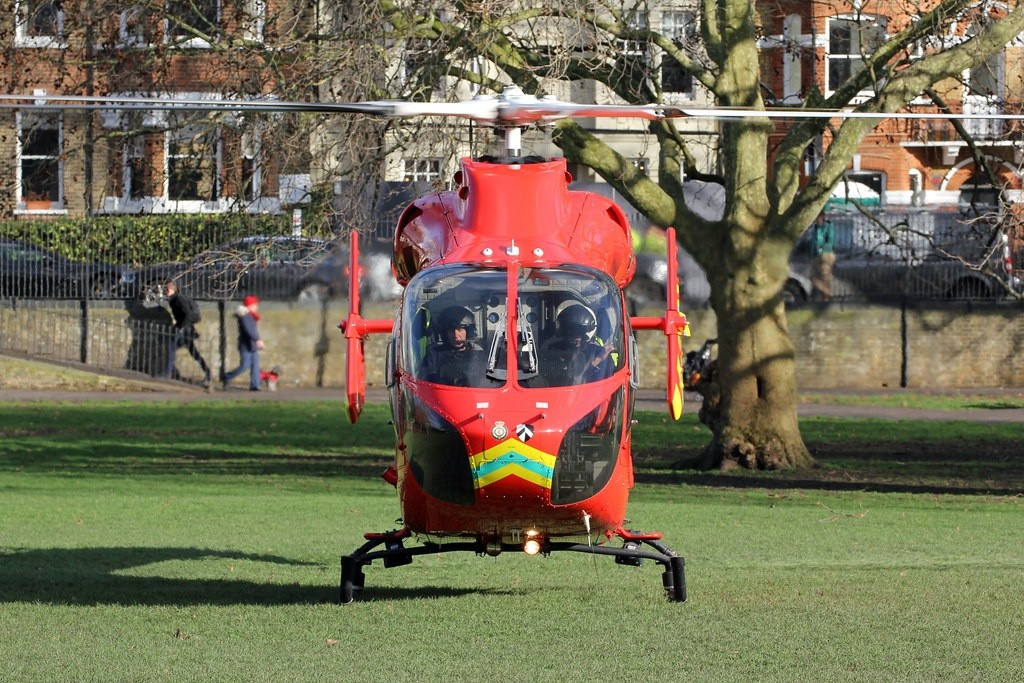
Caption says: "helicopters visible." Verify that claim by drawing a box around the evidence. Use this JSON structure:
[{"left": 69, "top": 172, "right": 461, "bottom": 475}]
[{"left": 0, "top": 85, "right": 1024, "bottom": 603}]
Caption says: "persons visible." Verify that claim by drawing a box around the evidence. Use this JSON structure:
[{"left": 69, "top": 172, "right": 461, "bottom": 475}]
[
  {"left": 417, "top": 305, "right": 491, "bottom": 431},
  {"left": 539, "top": 299, "right": 616, "bottom": 437},
  {"left": 164, "top": 282, "right": 213, "bottom": 388},
  {"left": 221, "top": 294, "right": 266, "bottom": 392}
]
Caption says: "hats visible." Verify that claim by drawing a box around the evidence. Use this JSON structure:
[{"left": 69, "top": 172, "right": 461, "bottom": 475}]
[{"left": 243, "top": 295, "right": 259, "bottom": 306}]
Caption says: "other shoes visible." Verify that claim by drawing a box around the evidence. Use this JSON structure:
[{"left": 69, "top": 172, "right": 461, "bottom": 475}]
[
  {"left": 250, "top": 388, "right": 262, "bottom": 392},
  {"left": 202, "top": 374, "right": 212, "bottom": 388},
  {"left": 222, "top": 373, "right": 230, "bottom": 391}
]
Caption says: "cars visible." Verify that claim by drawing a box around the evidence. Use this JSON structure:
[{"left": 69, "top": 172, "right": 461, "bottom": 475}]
[
  {"left": 116, "top": 236, "right": 372, "bottom": 306},
  {"left": 0, "top": 235, "right": 126, "bottom": 300}
]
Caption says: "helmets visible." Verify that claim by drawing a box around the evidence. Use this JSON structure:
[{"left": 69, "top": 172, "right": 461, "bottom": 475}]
[
  {"left": 558, "top": 305, "right": 595, "bottom": 334},
  {"left": 434, "top": 307, "right": 478, "bottom": 343}
]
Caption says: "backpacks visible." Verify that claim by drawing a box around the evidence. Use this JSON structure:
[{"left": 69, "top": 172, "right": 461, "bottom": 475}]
[{"left": 183, "top": 297, "right": 201, "bottom": 324}]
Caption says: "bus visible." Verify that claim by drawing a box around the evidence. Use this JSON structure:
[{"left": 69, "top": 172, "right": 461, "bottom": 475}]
[{"left": 783, "top": 204, "right": 1001, "bottom": 312}]
